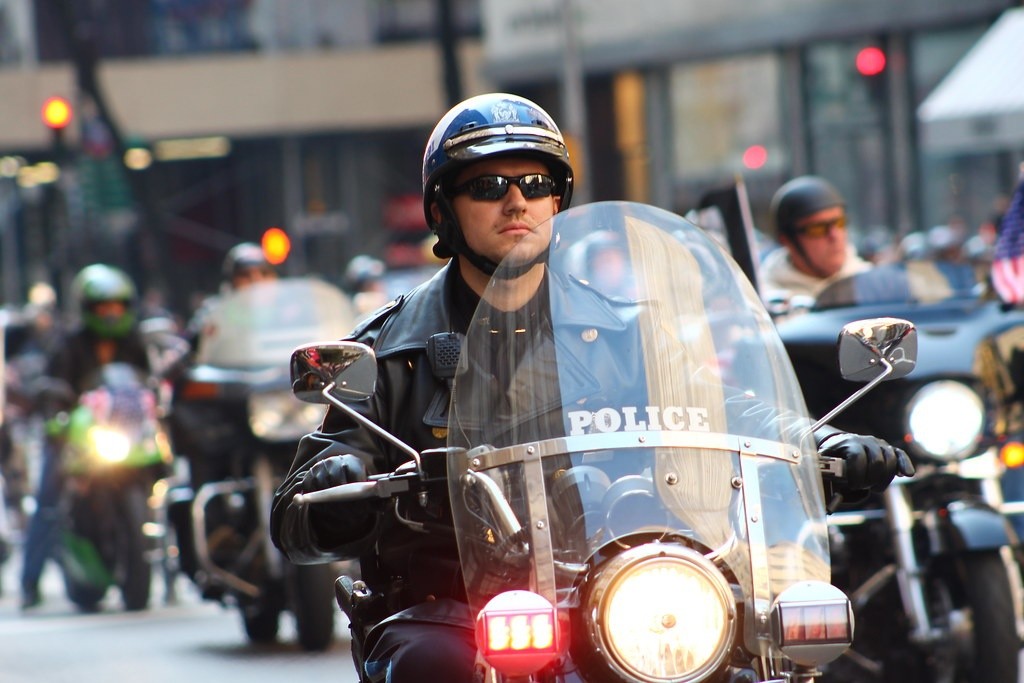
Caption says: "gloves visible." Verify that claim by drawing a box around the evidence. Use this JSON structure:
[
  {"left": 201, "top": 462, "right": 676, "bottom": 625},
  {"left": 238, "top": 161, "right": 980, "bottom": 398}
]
[
  {"left": 824, "top": 435, "right": 915, "bottom": 494},
  {"left": 302, "top": 453, "right": 368, "bottom": 492}
]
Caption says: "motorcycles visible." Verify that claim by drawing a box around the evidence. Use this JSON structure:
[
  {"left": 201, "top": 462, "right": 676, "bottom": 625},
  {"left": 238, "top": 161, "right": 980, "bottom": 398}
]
[{"left": 3, "top": 200, "right": 1024, "bottom": 683}]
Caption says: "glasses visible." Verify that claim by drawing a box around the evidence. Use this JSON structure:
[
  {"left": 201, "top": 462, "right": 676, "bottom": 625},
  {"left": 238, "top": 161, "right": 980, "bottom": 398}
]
[
  {"left": 448, "top": 173, "right": 556, "bottom": 201},
  {"left": 796, "top": 216, "right": 848, "bottom": 238}
]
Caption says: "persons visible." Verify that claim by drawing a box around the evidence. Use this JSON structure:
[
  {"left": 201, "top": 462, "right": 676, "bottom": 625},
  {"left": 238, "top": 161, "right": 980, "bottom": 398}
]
[
  {"left": 269, "top": 92, "right": 916, "bottom": 683},
  {"left": 0, "top": 173, "right": 1024, "bottom": 613}
]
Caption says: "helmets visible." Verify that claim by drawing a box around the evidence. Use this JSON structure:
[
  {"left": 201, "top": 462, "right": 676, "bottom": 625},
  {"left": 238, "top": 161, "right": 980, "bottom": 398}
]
[
  {"left": 77, "top": 263, "right": 135, "bottom": 336},
  {"left": 344, "top": 256, "right": 384, "bottom": 290},
  {"left": 421, "top": 92, "right": 575, "bottom": 234},
  {"left": 770, "top": 176, "right": 844, "bottom": 235},
  {"left": 226, "top": 242, "right": 270, "bottom": 277}
]
